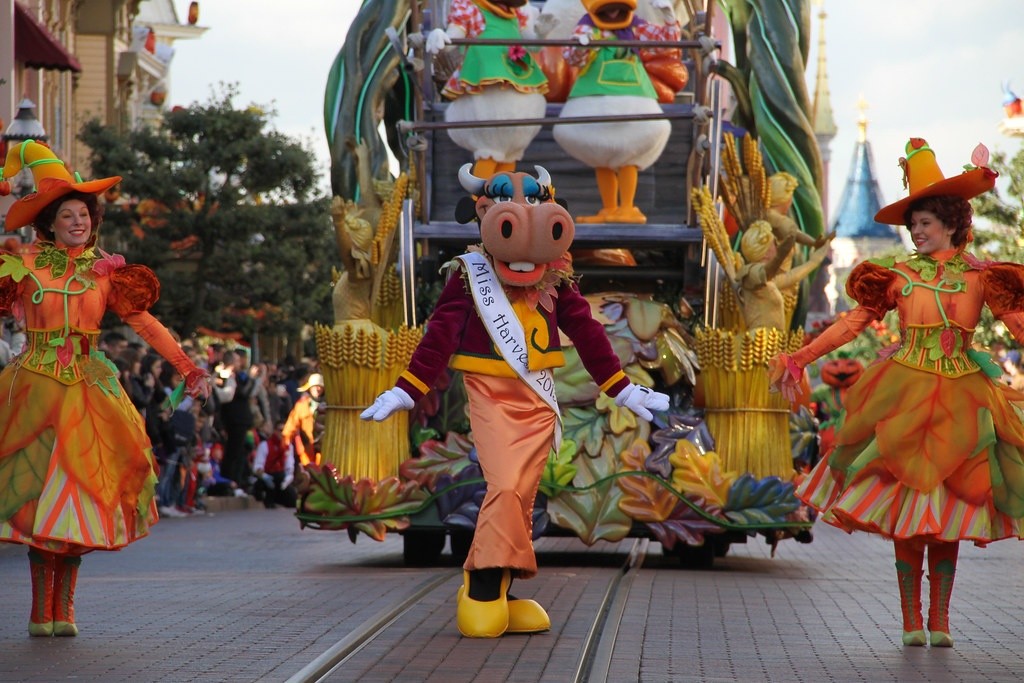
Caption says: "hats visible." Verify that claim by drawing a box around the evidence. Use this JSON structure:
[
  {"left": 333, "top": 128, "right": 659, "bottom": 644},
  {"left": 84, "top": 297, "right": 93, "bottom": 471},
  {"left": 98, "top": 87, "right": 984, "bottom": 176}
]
[
  {"left": 4, "top": 177, "right": 121, "bottom": 230},
  {"left": 874, "top": 168, "right": 996, "bottom": 223}
]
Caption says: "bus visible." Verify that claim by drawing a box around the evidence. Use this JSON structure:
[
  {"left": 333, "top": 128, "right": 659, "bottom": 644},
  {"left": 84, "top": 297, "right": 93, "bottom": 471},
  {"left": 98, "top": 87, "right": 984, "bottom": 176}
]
[{"left": 293, "top": 0, "right": 839, "bottom": 565}]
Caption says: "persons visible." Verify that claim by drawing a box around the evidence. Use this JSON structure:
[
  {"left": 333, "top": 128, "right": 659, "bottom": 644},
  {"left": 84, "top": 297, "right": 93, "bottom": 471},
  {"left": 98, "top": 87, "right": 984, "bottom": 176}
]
[
  {"left": 1, "top": 313, "right": 324, "bottom": 518},
  {"left": 0, "top": 139, "right": 216, "bottom": 636},
  {"left": 977, "top": 343, "right": 1024, "bottom": 391},
  {"left": 736, "top": 219, "right": 833, "bottom": 341},
  {"left": 802, "top": 398, "right": 819, "bottom": 471},
  {"left": 764, "top": 172, "right": 829, "bottom": 280},
  {"left": 769, "top": 136, "right": 1024, "bottom": 645},
  {"left": 308, "top": 193, "right": 377, "bottom": 326}
]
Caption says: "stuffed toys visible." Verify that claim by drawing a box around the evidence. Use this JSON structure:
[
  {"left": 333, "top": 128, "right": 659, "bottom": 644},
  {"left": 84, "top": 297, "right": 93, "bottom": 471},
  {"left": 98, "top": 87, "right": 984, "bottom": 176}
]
[
  {"left": 425, "top": 0, "right": 559, "bottom": 225},
  {"left": 552, "top": 2, "right": 682, "bottom": 223},
  {"left": 361, "top": 165, "right": 670, "bottom": 636}
]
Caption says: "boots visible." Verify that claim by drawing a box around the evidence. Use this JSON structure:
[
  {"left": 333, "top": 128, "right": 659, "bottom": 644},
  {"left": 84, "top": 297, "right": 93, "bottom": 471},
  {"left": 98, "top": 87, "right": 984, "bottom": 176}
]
[
  {"left": 926, "top": 539, "right": 958, "bottom": 647},
  {"left": 26, "top": 548, "right": 54, "bottom": 636},
  {"left": 54, "top": 553, "right": 82, "bottom": 635},
  {"left": 893, "top": 539, "right": 929, "bottom": 645}
]
